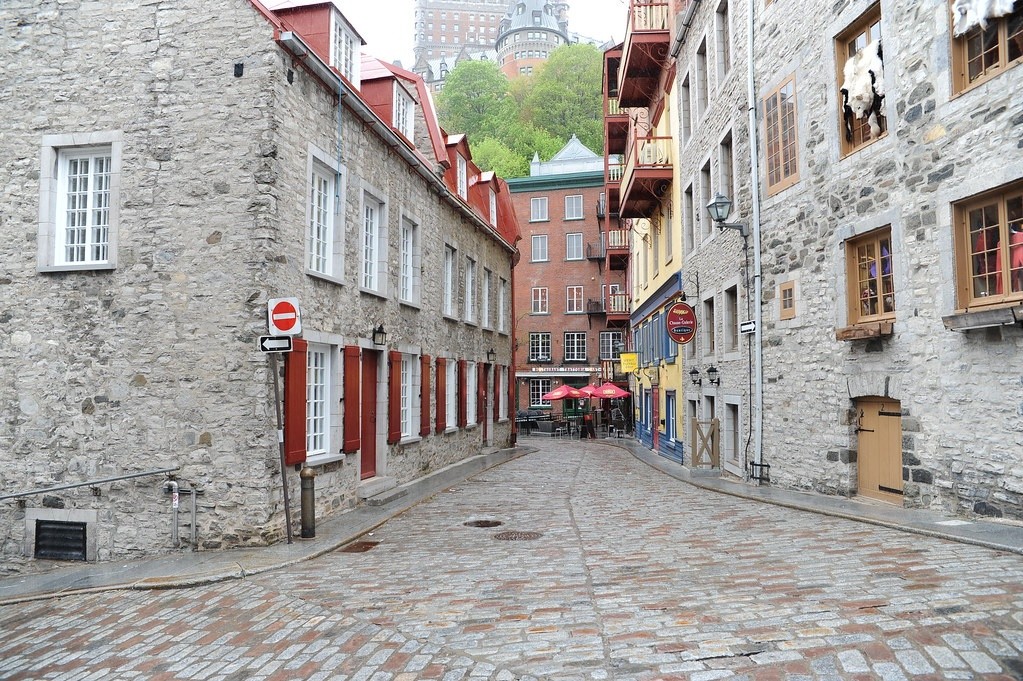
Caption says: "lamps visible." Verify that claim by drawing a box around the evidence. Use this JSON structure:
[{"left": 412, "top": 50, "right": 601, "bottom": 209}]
[
  {"left": 487, "top": 348, "right": 496, "bottom": 361},
  {"left": 706, "top": 192, "right": 750, "bottom": 237},
  {"left": 373, "top": 325, "right": 386, "bottom": 345}
]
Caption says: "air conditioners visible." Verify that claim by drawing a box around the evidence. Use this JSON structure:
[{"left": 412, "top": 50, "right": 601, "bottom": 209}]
[{"left": 641, "top": 143, "right": 658, "bottom": 168}]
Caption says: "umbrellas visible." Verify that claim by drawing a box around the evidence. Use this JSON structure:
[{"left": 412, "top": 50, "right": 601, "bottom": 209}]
[
  {"left": 544, "top": 384, "right": 589, "bottom": 416},
  {"left": 579, "top": 384, "right": 602, "bottom": 408},
  {"left": 591, "top": 382, "right": 630, "bottom": 411}
]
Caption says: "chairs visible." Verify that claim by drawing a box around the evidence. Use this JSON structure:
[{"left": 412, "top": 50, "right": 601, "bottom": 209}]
[{"left": 554, "top": 418, "right": 627, "bottom": 440}]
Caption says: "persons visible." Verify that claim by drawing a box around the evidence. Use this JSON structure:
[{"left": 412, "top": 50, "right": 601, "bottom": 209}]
[{"left": 611, "top": 405, "right": 625, "bottom": 438}]
